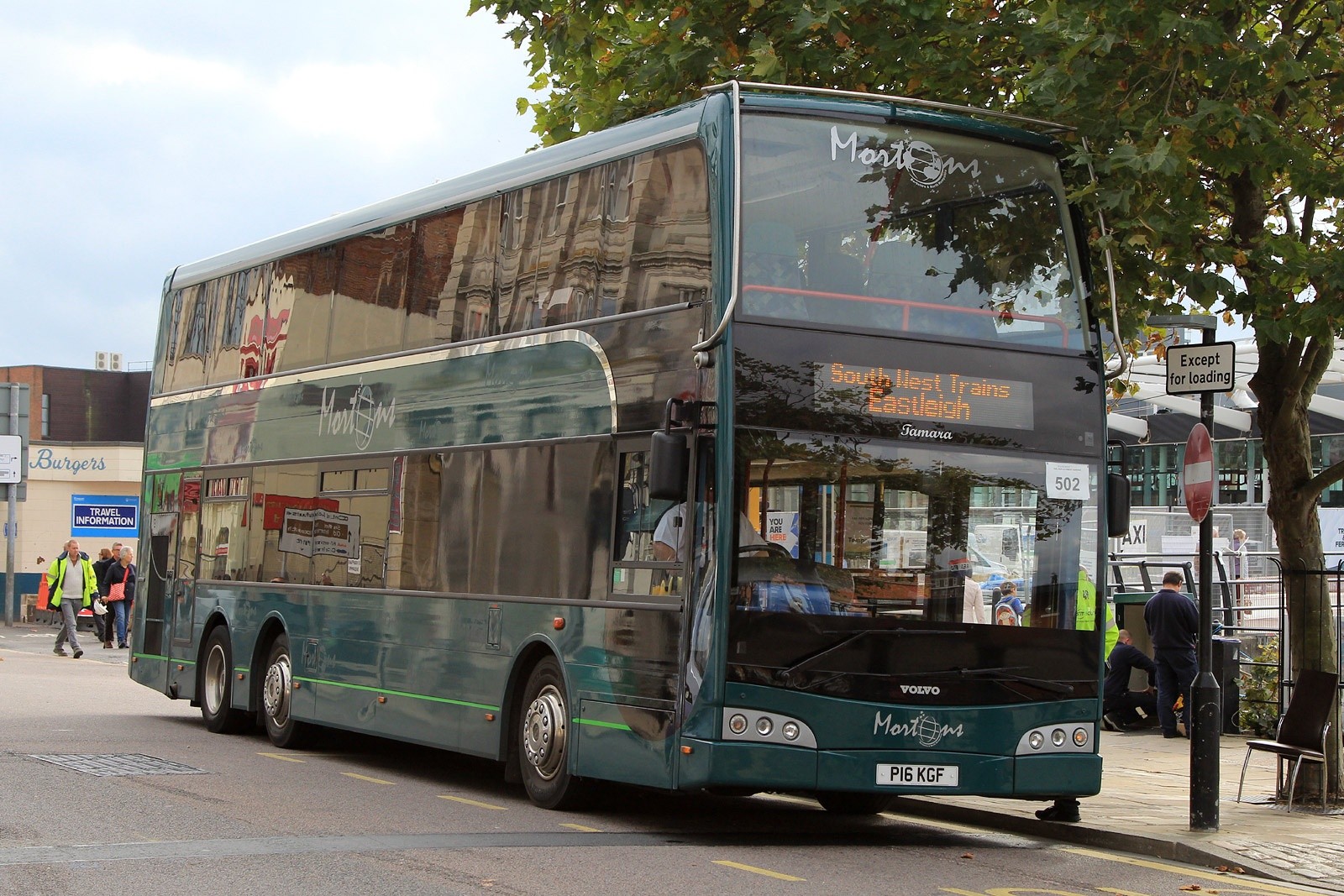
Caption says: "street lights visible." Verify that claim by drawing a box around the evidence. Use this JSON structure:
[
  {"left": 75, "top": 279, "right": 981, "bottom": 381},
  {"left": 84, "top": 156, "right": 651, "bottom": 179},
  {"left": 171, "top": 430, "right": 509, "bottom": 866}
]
[{"left": 1147, "top": 314, "right": 1221, "bottom": 831}]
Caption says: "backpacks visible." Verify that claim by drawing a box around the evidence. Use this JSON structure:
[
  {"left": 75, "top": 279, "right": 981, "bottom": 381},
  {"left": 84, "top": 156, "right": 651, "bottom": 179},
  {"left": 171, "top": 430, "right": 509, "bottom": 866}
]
[{"left": 995, "top": 597, "right": 1017, "bottom": 626}]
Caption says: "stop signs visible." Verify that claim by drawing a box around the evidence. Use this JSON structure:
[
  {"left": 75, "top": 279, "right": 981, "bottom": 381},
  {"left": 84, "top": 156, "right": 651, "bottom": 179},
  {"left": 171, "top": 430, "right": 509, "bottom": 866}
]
[{"left": 1183, "top": 424, "right": 1214, "bottom": 522}]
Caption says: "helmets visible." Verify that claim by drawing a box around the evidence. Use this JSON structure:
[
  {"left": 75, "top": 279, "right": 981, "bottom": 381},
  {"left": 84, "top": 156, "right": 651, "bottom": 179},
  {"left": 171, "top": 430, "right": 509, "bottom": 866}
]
[{"left": 93, "top": 599, "right": 108, "bottom": 615}]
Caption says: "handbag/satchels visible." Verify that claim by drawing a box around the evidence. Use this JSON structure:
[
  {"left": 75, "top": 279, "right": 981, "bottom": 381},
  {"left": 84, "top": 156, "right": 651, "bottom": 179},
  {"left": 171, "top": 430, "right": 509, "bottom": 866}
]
[
  {"left": 107, "top": 583, "right": 125, "bottom": 601},
  {"left": 1244, "top": 587, "right": 1252, "bottom": 615}
]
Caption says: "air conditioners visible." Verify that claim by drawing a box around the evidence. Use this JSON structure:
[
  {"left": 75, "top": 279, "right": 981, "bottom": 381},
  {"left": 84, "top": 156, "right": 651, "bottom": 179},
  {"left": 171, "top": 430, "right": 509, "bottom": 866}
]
[
  {"left": 111, "top": 352, "right": 122, "bottom": 371},
  {"left": 96, "top": 351, "right": 108, "bottom": 370}
]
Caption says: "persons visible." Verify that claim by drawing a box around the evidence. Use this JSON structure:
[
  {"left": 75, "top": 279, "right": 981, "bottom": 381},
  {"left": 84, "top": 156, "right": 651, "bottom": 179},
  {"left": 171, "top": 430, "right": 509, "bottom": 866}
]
[
  {"left": 1233, "top": 530, "right": 1248, "bottom": 627},
  {"left": 1143, "top": 572, "right": 1200, "bottom": 740},
  {"left": 963, "top": 559, "right": 985, "bottom": 624},
  {"left": 1076, "top": 563, "right": 1119, "bottom": 661},
  {"left": 92, "top": 542, "right": 137, "bottom": 648},
  {"left": 1193, "top": 527, "right": 1218, "bottom": 583},
  {"left": 46, "top": 540, "right": 100, "bottom": 658},
  {"left": 652, "top": 468, "right": 770, "bottom": 575},
  {"left": 1101, "top": 629, "right": 1156, "bottom": 733},
  {"left": 995, "top": 582, "right": 1024, "bottom": 627}
]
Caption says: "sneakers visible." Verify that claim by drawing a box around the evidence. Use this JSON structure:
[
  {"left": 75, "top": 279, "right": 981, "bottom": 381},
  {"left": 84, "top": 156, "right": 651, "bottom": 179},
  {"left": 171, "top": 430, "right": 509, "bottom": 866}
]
[
  {"left": 73, "top": 649, "right": 83, "bottom": 659},
  {"left": 1100, "top": 711, "right": 1128, "bottom": 732},
  {"left": 1035, "top": 805, "right": 1081, "bottom": 822},
  {"left": 118, "top": 641, "right": 129, "bottom": 648},
  {"left": 1163, "top": 729, "right": 1190, "bottom": 739},
  {"left": 53, "top": 648, "right": 68, "bottom": 657},
  {"left": 103, "top": 641, "right": 113, "bottom": 649}
]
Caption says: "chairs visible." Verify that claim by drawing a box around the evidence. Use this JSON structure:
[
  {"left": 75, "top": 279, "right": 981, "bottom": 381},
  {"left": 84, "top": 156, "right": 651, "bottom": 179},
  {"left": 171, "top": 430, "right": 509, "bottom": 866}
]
[{"left": 1237, "top": 667, "right": 1339, "bottom": 813}]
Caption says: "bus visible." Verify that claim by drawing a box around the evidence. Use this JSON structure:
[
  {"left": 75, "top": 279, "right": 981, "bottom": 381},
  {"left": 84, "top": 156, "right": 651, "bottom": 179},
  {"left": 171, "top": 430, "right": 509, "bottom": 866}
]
[{"left": 126, "top": 79, "right": 1132, "bottom": 813}]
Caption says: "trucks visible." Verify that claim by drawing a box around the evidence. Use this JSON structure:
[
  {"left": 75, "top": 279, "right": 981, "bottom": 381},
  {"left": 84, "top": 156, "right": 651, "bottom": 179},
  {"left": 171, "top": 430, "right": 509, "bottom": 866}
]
[{"left": 880, "top": 529, "right": 1008, "bottom": 582}]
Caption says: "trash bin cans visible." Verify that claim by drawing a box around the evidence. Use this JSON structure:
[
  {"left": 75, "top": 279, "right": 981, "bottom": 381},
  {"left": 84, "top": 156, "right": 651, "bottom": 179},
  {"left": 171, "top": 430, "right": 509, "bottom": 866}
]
[{"left": 1196, "top": 638, "right": 1243, "bottom": 734}]
